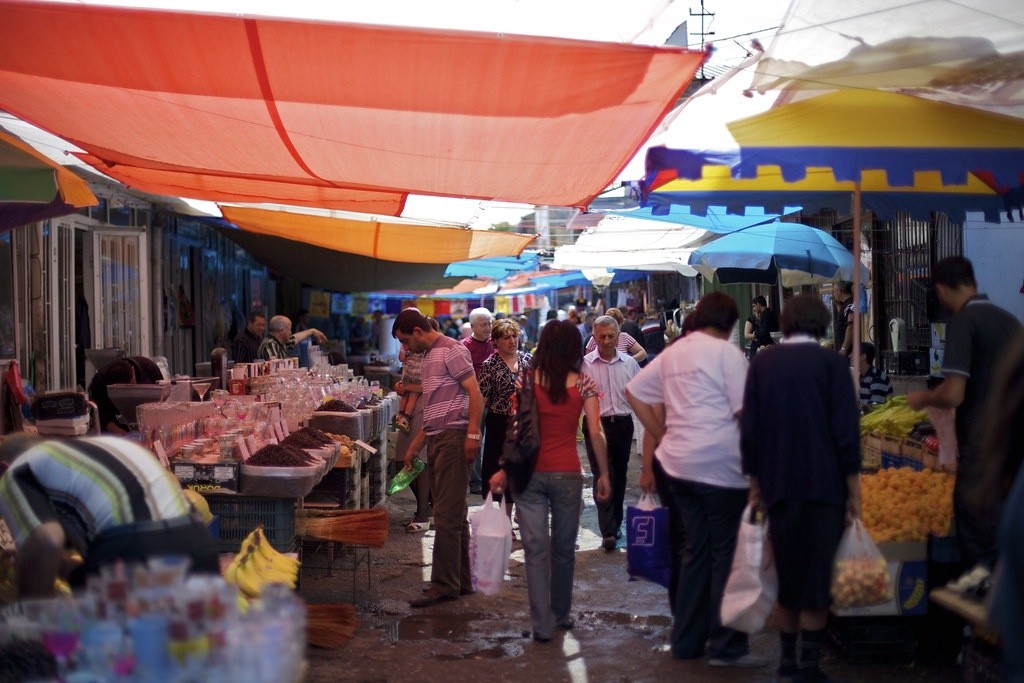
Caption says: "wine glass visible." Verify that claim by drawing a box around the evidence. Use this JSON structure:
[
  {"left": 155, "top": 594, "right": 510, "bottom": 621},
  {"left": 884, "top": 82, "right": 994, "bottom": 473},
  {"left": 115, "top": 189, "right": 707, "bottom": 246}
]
[
  {"left": 236, "top": 406, "right": 249, "bottom": 427},
  {"left": 42, "top": 609, "right": 79, "bottom": 682},
  {"left": 192, "top": 383, "right": 211, "bottom": 402}
]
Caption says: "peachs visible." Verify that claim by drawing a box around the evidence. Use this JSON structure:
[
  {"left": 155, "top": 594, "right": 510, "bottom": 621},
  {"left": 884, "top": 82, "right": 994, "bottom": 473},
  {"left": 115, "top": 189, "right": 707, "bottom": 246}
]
[{"left": 855, "top": 465, "right": 956, "bottom": 542}]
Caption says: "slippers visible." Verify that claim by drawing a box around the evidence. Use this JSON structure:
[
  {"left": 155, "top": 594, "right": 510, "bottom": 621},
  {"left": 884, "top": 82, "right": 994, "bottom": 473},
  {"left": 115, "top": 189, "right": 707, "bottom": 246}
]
[
  {"left": 407, "top": 519, "right": 430, "bottom": 532},
  {"left": 429, "top": 516, "right": 435, "bottom": 525}
]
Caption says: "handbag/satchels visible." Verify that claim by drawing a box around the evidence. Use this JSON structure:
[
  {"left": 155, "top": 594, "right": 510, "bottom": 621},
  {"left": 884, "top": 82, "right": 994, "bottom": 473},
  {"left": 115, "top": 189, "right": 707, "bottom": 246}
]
[
  {"left": 625, "top": 489, "right": 670, "bottom": 589},
  {"left": 831, "top": 516, "right": 892, "bottom": 605},
  {"left": 471, "top": 487, "right": 513, "bottom": 596},
  {"left": 719, "top": 501, "right": 779, "bottom": 634},
  {"left": 923, "top": 401, "right": 958, "bottom": 465},
  {"left": 502, "top": 366, "right": 539, "bottom": 493}
]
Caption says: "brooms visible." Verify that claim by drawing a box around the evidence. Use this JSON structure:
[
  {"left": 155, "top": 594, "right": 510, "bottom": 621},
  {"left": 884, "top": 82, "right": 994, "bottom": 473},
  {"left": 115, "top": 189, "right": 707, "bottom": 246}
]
[
  {"left": 294, "top": 507, "right": 389, "bottom": 548},
  {"left": 305, "top": 603, "right": 361, "bottom": 649}
]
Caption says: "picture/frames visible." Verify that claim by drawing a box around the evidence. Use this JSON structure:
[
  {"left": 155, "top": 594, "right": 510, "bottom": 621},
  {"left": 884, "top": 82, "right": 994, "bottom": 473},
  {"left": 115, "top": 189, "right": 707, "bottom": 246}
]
[{"left": 249, "top": 269, "right": 265, "bottom": 317}]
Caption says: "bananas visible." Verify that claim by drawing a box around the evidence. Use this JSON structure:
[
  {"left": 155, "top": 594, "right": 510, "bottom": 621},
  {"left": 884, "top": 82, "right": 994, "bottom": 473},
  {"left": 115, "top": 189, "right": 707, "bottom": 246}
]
[{"left": 226, "top": 523, "right": 301, "bottom": 598}]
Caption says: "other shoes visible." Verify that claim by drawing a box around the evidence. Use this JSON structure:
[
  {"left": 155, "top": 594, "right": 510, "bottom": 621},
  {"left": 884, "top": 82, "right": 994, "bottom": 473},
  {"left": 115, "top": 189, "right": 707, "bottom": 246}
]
[
  {"left": 599, "top": 527, "right": 622, "bottom": 551},
  {"left": 409, "top": 587, "right": 459, "bottom": 605},
  {"left": 470, "top": 488, "right": 482, "bottom": 495},
  {"left": 560, "top": 617, "right": 575, "bottom": 628},
  {"left": 708, "top": 651, "right": 767, "bottom": 668}
]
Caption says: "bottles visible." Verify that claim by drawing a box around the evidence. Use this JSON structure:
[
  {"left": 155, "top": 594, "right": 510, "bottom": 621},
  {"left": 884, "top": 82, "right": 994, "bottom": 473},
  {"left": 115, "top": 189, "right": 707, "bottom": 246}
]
[
  {"left": 370, "top": 352, "right": 377, "bottom": 365},
  {"left": 86, "top": 603, "right": 123, "bottom": 683},
  {"left": 386, "top": 458, "right": 426, "bottom": 498}
]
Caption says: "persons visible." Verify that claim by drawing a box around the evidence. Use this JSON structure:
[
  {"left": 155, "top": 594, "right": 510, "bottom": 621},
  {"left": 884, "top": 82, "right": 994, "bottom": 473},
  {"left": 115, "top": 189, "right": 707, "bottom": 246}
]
[
  {"left": 744, "top": 296, "right": 778, "bottom": 361},
  {"left": 392, "top": 307, "right": 487, "bottom": 604},
  {"left": 982, "top": 333, "right": 1024, "bottom": 683},
  {"left": 626, "top": 291, "right": 751, "bottom": 659},
  {"left": 740, "top": 297, "right": 861, "bottom": 683},
  {"left": 849, "top": 343, "right": 893, "bottom": 409},
  {"left": 830, "top": 283, "right": 854, "bottom": 355},
  {"left": 236, "top": 299, "right": 696, "bottom": 551},
  {"left": 0, "top": 432, "right": 220, "bottom": 578},
  {"left": 911, "top": 256, "right": 1024, "bottom": 590},
  {"left": 491, "top": 320, "right": 611, "bottom": 641}
]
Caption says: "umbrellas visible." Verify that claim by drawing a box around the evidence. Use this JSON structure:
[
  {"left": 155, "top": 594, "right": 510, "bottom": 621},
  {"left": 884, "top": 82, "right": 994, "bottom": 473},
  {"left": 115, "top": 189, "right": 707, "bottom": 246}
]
[
  {"left": 0, "top": 125, "right": 100, "bottom": 229},
  {"left": 638, "top": 89, "right": 1024, "bottom": 396},
  {"left": 688, "top": 218, "right": 869, "bottom": 314}
]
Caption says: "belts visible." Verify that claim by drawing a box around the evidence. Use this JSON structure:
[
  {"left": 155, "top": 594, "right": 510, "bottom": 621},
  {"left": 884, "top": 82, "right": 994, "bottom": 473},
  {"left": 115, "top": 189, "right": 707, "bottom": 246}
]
[{"left": 94, "top": 512, "right": 204, "bottom": 543}]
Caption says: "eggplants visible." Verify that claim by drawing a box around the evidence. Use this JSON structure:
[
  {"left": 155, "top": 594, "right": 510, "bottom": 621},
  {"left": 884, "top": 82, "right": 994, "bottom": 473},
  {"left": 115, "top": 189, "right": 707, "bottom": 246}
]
[{"left": 907, "top": 422, "right": 935, "bottom": 446}]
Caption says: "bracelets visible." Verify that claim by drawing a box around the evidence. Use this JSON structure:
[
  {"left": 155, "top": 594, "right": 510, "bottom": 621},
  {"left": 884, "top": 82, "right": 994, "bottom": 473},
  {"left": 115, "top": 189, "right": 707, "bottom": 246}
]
[
  {"left": 467, "top": 433, "right": 480, "bottom": 440},
  {"left": 841, "top": 347, "right": 845, "bottom": 351},
  {"left": 983, "top": 579, "right": 992, "bottom": 591}
]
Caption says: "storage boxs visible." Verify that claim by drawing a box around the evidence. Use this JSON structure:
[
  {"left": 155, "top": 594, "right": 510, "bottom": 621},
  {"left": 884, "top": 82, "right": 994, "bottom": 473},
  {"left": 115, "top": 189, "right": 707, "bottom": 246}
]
[
  {"left": 879, "top": 276, "right": 949, "bottom": 377},
  {"left": 387, "top": 431, "right": 400, "bottom": 459},
  {"left": 862, "top": 444, "right": 925, "bottom": 475},
  {"left": 201, "top": 493, "right": 298, "bottom": 553},
  {"left": 819, "top": 536, "right": 927, "bottom": 667}
]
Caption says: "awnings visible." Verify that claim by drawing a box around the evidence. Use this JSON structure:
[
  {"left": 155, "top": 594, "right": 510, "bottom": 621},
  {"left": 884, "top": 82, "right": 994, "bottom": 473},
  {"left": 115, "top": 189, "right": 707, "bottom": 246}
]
[{"left": 0, "top": 0, "right": 1024, "bottom": 294}]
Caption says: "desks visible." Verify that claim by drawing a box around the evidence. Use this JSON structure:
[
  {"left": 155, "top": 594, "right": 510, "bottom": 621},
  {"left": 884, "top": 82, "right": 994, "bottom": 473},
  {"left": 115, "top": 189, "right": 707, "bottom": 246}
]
[{"left": 365, "top": 372, "right": 391, "bottom": 389}]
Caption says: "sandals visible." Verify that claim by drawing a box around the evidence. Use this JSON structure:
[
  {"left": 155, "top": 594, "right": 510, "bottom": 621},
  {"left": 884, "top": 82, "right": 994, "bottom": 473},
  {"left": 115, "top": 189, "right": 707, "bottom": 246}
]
[{"left": 393, "top": 413, "right": 410, "bottom": 434}]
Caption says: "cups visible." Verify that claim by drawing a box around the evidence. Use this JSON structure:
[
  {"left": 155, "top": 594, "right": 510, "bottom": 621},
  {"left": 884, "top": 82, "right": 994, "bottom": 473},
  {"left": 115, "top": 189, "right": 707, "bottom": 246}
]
[
  {"left": 147, "top": 556, "right": 188, "bottom": 588},
  {"left": 128, "top": 616, "right": 167, "bottom": 682},
  {"left": 180, "top": 438, "right": 214, "bottom": 458},
  {"left": 211, "top": 390, "right": 227, "bottom": 411},
  {"left": 217, "top": 429, "right": 245, "bottom": 459}
]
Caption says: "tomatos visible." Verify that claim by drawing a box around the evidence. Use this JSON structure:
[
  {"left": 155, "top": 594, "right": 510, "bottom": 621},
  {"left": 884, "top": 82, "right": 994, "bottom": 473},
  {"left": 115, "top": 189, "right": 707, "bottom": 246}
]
[{"left": 925, "top": 435, "right": 939, "bottom": 453}]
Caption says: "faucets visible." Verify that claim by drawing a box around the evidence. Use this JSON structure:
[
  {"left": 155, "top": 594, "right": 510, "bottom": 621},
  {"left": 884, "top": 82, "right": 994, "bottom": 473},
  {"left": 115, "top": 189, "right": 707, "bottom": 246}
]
[{"left": 84, "top": 398, "right": 100, "bottom": 437}]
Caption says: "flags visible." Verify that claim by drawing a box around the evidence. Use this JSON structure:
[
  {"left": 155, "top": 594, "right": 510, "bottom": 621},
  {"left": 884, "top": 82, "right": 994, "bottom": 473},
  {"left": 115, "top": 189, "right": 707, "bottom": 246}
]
[{"left": 309, "top": 292, "right": 524, "bottom": 318}]
